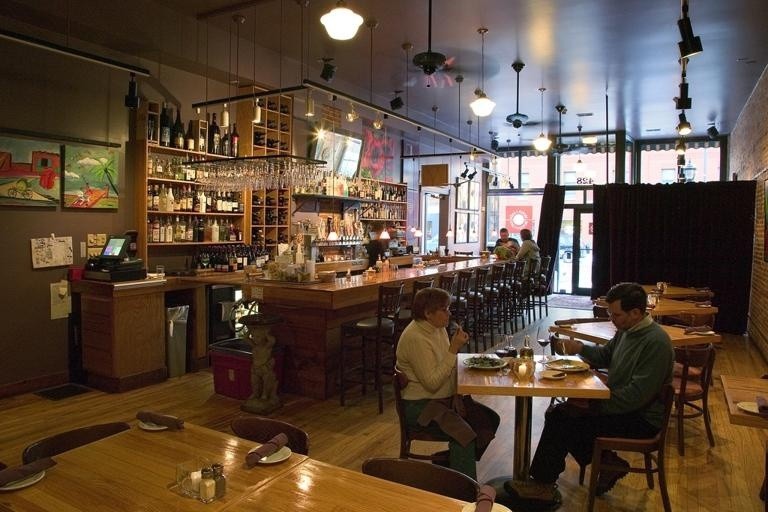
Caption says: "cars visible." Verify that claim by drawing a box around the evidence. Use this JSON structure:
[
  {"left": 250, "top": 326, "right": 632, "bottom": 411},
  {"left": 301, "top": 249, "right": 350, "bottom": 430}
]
[{"left": 558, "top": 229, "right": 591, "bottom": 263}]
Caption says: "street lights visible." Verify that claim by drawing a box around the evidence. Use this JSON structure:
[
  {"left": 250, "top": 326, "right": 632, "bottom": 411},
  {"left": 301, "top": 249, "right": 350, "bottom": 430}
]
[{"left": 683, "top": 159, "right": 696, "bottom": 182}]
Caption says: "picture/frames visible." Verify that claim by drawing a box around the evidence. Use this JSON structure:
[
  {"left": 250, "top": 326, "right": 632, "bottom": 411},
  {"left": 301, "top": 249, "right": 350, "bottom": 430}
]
[{"left": 455, "top": 177, "right": 480, "bottom": 244}]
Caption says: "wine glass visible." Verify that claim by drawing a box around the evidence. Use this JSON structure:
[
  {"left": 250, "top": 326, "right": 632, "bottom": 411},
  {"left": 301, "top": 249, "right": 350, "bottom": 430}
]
[
  {"left": 196, "top": 158, "right": 323, "bottom": 190},
  {"left": 494, "top": 333, "right": 507, "bottom": 376},
  {"left": 505, "top": 335, "right": 516, "bottom": 357},
  {"left": 535, "top": 326, "right": 552, "bottom": 364},
  {"left": 202, "top": 253, "right": 210, "bottom": 277}
]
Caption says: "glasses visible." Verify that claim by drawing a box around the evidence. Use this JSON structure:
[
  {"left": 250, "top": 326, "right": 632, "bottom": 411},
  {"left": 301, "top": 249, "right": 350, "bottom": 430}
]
[{"left": 607, "top": 308, "right": 630, "bottom": 319}]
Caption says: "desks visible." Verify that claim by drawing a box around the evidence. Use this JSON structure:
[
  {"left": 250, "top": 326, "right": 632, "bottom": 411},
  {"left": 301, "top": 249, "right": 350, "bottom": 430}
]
[
  {"left": 0, "top": 415, "right": 483, "bottom": 512},
  {"left": 719, "top": 373, "right": 767, "bottom": 429}
]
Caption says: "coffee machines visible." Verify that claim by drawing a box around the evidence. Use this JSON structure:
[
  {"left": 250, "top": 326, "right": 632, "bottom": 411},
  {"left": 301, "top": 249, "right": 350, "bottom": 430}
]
[{"left": 304, "top": 234, "right": 362, "bottom": 261}]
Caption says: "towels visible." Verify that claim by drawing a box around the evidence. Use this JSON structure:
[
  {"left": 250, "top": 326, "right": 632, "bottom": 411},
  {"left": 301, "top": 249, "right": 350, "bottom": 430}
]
[
  {"left": 0, "top": 456, "right": 57, "bottom": 487},
  {"left": 475, "top": 484, "right": 497, "bottom": 512},
  {"left": 246, "top": 432, "right": 290, "bottom": 468},
  {"left": 134, "top": 410, "right": 184, "bottom": 433},
  {"left": 754, "top": 394, "right": 767, "bottom": 412}
]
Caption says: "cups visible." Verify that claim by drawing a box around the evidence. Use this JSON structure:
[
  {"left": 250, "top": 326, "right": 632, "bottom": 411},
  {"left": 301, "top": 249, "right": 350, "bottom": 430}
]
[
  {"left": 444, "top": 248, "right": 449, "bottom": 256},
  {"left": 156, "top": 265, "right": 164, "bottom": 279},
  {"left": 647, "top": 281, "right": 667, "bottom": 308}
]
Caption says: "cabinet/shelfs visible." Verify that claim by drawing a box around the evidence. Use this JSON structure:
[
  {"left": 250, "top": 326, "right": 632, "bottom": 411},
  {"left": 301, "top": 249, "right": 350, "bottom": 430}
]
[
  {"left": 343, "top": 177, "right": 407, "bottom": 248},
  {"left": 292, "top": 193, "right": 380, "bottom": 216},
  {"left": 236, "top": 86, "right": 293, "bottom": 259},
  {"left": 125, "top": 140, "right": 245, "bottom": 272}
]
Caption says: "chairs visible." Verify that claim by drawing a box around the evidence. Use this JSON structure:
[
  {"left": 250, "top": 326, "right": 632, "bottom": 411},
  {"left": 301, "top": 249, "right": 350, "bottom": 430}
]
[
  {"left": 230, "top": 416, "right": 309, "bottom": 456},
  {"left": 394, "top": 283, "right": 721, "bottom": 512},
  {"left": 340, "top": 255, "right": 552, "bottom": 413},
  {"left": 23, "top": 421, "right": 130, "bottom": 464},
  {"left": 361, "top": 456, "right": 480, "bottom": 504}
]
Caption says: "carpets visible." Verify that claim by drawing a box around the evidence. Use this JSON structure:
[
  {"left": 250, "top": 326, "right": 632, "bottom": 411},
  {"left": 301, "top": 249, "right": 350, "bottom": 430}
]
[{"left": 33, "top": 384, "right": 93, "bottom": 401}]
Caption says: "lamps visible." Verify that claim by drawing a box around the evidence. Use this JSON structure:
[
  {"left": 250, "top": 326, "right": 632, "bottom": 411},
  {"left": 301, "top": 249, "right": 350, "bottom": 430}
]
[
  {"left": 460, "top": 161, "right": 515, "bottom": 190},
  {"left": 670, "top": 0, "right": 703, "bottom": 182},
  {"left": 123, "top": 70, "right": 140, "bottom": 114},
  {"left": 326, "top": 91, "right": 454, "bottom": 243},
  {"left": 217, "top": 0, "right": 584, "bottom": 168}
]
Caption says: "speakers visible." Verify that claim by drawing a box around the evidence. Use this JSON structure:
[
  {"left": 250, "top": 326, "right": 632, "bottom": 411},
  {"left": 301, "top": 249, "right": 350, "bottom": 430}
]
[
  {"left": 708, "top": 127, "right": 719, "bottom": 139},
  {"left": 492, "top": 139, "right": 498, "bottom": 150},
  {"left": 320, "top": 63, "right": 336, "bottom": 82},
  {"left": 390, "top": 97, "right": 404, "bottom": 111}
]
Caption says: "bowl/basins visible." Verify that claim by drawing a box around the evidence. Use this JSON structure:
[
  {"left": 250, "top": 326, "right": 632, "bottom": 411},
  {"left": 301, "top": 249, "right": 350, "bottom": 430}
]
[
  {"left": 318, "top": 270, "right": 337, "bottom": 283},
  {"left": 479, "top": 251, "right": 491, "bottom": 258}
]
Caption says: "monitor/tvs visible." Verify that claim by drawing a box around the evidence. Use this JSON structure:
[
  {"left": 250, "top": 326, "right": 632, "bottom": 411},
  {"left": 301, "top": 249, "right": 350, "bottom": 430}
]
[
  {"left": 310, "top": 125, "right": 364, "bottom": 178},
  {"left": 99, "top": 235, "right": 130, "bottom": 258}
]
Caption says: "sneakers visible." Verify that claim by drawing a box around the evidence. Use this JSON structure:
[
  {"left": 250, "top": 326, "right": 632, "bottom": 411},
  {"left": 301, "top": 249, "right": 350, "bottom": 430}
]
[
  {"left": 431, "top": 451, "right": 447, "bottom": 468},
  {"left": 504, "top": 478, "right": 560, "bottom": 502},
  {"left": 595, "top": 452, "right": 631, "bottom": 497}
]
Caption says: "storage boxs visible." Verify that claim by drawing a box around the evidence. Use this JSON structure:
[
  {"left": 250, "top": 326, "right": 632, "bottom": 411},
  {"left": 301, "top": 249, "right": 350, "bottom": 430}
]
[{"left": 211, "top": 337, "right": 288, "bottom": 402}]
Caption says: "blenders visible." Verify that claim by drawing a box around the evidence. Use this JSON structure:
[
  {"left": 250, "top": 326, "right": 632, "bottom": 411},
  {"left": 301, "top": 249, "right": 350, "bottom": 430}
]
[{"left": 388, "top": 230, "right": 408, "bottom": 256}]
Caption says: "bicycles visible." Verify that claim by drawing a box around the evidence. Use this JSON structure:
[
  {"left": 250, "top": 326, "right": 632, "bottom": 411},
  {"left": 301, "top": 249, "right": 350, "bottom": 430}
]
[{"left": 7, "top": 186, "right": 33, "bottom": 200}]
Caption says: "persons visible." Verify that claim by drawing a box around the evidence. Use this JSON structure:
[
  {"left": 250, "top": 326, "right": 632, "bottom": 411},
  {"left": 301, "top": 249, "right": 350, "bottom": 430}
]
[
  {"left": 365, "top": 224, "right": 390, "bottom": 273},
  {"left": 394, "top": 286, "right": 501, "bottom": 482},
  {"left": 504, "top": 282, "right": 675, "bottom": 503},
  {"left": 510, "top": 229, "right": 541, "bottom": 277},
  {"left": 495, "top": 227, "right": 520, "bottom": 255},
  {"left": 243, "top": 323, "right": 278, "bottom": 402}
]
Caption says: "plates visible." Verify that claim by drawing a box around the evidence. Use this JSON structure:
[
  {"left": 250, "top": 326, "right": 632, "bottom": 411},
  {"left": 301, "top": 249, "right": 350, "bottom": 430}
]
[
  {"left": 138, "top": 415, "right": 175, "bottom": 430},
  {"left": 461, "top": 502, "right": 513, "bottom": 512},
  {"left": 248, "top": 443, "right": 291, "bottom": 464},
  {"left": 737, "top": 401, "right": 767, "bottom": 415},
  {"left": 546, "top": 360, "right": 591, "bottom": 372},
  {"left": 539, "top": 370, "right": 568, "bottom": 380},
  {"left": 1, "top": 468, "right": 47, "bottom": 492},
  {"left": 463, "top": 358, "right": 508, "bottom": 370}
]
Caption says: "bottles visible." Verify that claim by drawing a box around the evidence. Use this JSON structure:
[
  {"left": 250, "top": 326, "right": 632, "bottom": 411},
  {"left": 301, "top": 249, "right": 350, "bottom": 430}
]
[
  {"left": 146, "top": 183, "right": 245, "bottom": 213},
  {"left": 198, "top": 467, "right": 217, "bottom": 505},
  {"left": 147, "top": 215, "right": 243, "bottom": 243},
  {"left": 147, "top": 116, "right": 157, "bottom": 142},
  {"left": 190, "top": 244, "right": 271, "bottom": 272},
  {"left": 317, "top": 170, "right": 404, "bottom": 201},
  {"left": 253, "top": 97, "right": 288, "bottom": 151},
  {"left": 253, "top": 195, "right": 287, "bottom": 244},
  {"left": 147, "top": 146, "right": 209, "bottom": 181},
  {"left": 519, "top": 333, "right": 534, "bottom": 359},
  {"left": 159, "top": 101, "right": 240, "bottom": 158},
  {"left": 362, "top": 269, "right": 377, "bottom": 280},
  {"left": 359, "top": 204, "right": 405, "bottom": 219},
  {"left": 211, "top": 463, "right": 225, "bottom": 502},
  {"left": 376, "top": 254, "right": 399, "bottom": 274}
]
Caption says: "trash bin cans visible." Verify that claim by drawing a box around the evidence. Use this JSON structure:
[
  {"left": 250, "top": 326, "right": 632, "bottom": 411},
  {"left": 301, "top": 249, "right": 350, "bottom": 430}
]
[{"left": 166, "top": 304, "right": 190, "bottom": 378}]
